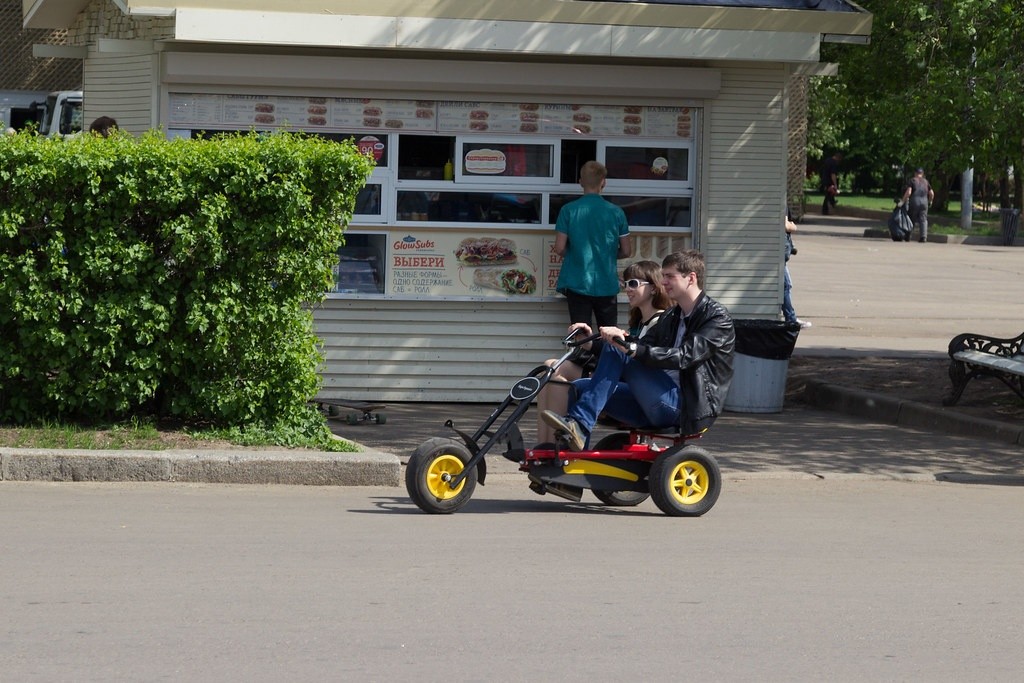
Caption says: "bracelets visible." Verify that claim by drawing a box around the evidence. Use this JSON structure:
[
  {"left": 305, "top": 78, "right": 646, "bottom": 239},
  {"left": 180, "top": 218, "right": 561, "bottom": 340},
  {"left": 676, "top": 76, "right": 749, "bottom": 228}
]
[{"left": 625, "top": 350, "right": 634, "bottom": 357}]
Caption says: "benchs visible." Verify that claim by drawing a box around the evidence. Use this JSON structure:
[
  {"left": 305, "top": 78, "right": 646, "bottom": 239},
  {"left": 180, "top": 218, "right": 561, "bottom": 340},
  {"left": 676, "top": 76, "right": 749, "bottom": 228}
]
[{"left": 944, "top": 332, "right": 1024, "bottom": 406}]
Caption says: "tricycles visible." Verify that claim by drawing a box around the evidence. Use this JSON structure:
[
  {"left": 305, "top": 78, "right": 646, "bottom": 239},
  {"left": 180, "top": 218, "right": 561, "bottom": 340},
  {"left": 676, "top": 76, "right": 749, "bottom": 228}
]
[{"left": 405, "top": 324, "right": 722, "bottom": 516}]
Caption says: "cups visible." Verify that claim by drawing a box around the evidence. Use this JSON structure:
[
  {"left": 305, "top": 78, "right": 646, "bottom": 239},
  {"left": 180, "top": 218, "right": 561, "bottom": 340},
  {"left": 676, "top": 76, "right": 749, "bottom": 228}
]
[{"left": 398, "top": 212, "right": 428, "bottom": 220}]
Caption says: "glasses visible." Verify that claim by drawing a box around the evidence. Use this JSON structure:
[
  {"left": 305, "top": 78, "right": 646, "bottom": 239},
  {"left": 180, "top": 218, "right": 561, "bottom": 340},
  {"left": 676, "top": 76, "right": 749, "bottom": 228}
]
[{"left": 624, "top": 278, "right": 650, "bottom": 291}]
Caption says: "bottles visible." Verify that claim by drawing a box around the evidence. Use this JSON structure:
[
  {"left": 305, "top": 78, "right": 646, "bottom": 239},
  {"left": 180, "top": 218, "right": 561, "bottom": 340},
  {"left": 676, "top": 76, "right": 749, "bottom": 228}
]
[{"left": 444, "top": 159, "right": 453, "bottom": 180}]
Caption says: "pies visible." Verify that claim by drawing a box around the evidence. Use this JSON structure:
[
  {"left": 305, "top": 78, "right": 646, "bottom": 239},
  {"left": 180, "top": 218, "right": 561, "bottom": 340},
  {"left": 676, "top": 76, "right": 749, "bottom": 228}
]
[{"left": 473, "top": 268, "right": 536, "bottom": 295}]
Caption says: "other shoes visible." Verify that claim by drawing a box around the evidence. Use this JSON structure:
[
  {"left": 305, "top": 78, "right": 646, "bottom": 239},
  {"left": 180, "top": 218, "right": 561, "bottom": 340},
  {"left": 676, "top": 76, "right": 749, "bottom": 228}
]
[
  {"left": 903, "top": 233, "right": 911, "bottom": 242},
  {"left": 918, "top": 237, "right": 927, "bottom": 243},
  {"left": 823, "top": 213, "right": 832, "bottom": 215},
  {"left": 796, "top": 317, "right": 812, "bottom": 328},
  {"left": 833, "top": 199, "right": 838, "bottom": 207}
]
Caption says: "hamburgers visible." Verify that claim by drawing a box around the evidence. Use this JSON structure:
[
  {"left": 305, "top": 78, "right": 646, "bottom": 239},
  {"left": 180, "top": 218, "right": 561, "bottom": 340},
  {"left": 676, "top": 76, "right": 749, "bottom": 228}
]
[{"left": 454, "top": 237, "right": 517, "bottom": 266}]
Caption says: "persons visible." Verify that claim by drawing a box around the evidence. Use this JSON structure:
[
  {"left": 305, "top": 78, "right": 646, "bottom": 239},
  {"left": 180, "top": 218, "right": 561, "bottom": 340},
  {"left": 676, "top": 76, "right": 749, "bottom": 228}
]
[
  {"left": 88, "top": 115, "right": 119, "bottom": 139},
  {"left": 820, "top": 152, "right": 844, "bottom": 216},
  {"left": 982, "top": 169, "right": 995, "bottom": 213},
  {"left": 540, "top": 251, "right": 735, "bottom": 451},
  {"left": 554, "top": 161, "right": 632, "bottom": 334},
  {"left": 534, "top": 260, "right": 676, "bottom": 451},
  {"left": 784, "top": 203, "right": 809, "bottom": 329},
  {"left": 898, "top": 166, "right": 934, "bottom": 243}
]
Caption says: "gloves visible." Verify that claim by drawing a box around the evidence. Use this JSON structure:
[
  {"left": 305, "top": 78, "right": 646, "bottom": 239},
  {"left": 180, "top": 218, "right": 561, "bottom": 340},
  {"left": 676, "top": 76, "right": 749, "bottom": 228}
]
[
  {"left": 897, "top": 200, "right": 904, "bottom": 208},
  {"left": 928, "top": 201, "right": 932, "bottom": 208}
]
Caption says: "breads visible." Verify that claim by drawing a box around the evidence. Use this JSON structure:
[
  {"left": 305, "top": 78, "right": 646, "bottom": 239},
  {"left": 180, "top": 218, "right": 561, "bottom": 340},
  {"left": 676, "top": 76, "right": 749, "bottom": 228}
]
[{"left": 630, "top": 235, "right": 686, "bottom": 258}]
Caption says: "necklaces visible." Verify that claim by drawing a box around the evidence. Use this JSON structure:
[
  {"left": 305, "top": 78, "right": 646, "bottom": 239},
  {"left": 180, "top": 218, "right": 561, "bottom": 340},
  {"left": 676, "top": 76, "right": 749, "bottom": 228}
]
[{"left": 635, "top": 311, "right": 658, "bottom": 338}]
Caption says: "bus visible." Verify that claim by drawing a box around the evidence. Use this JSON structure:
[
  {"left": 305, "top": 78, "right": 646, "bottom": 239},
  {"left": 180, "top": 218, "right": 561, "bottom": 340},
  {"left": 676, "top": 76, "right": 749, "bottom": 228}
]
[{"left": 30, "top": 90, "right": 83, "bottom": 138}]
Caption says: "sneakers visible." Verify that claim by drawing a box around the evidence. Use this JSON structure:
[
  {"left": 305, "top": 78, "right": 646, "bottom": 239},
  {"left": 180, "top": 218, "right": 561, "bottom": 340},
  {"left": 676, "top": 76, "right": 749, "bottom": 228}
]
[
  {"left": 541, "top": 409, "right": 588, "bottom": 451},
  {"left": 528, "top": 472, "right": 584, "bottom": 503}
]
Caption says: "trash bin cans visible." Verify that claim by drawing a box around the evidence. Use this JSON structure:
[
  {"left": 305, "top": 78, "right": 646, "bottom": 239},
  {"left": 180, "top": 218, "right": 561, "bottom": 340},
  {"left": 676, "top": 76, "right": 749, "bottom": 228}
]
[
  {"left": 723, "top": 318, "right": 801, "bottom": 413},
  {"left": 1000, "top": 209, "right": 1020, "bottom": 246}
]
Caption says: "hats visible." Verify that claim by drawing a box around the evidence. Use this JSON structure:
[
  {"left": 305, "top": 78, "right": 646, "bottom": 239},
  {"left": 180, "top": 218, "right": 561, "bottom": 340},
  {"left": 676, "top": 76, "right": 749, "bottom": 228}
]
[
  {"left": 914, "top": 166, "right": 924, "bottom": 173},
  {"left": 834, "top": 152, "right": 843, "bottom": 158}
]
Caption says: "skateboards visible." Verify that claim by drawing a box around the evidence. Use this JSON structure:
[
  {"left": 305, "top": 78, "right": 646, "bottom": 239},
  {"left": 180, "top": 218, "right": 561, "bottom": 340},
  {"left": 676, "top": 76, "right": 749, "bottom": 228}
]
[{"left": 309, "top": 398, "right": 387, "bottom": 425}]
